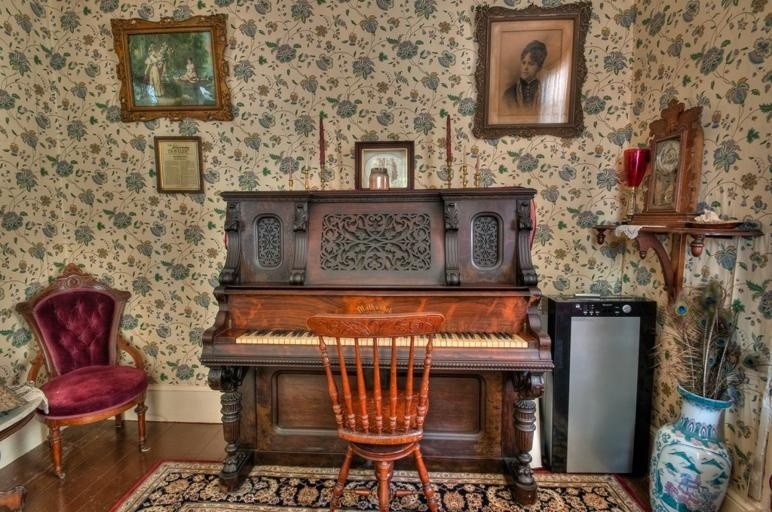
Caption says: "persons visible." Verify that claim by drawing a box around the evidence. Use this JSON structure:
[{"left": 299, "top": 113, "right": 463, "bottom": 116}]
[
  {"left": 178, "top": 55, "right": 199, "bottom": 83},
  {"left": 144, "top": 44, "right": 168, "bottom": 97},
  {"left": 502, "top": 39, "right": 552, "bottom": 114}
]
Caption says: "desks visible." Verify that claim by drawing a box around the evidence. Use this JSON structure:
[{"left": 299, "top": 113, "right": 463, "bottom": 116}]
[{"left": 0, "top": 381, "right": 44, "bottom": 512}]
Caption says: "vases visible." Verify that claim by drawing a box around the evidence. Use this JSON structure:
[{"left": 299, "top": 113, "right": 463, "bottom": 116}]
[{"left": 647, "top": 381, "right": 737, "bottom": 512}]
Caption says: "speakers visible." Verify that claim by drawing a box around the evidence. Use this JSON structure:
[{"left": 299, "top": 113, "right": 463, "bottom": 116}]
[{"left": 541, "top": 293, "right": 656, "bottom": 476}]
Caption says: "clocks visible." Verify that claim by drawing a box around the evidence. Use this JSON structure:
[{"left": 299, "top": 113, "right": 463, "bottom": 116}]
[{"left": 641, "top": 97, "right": 707, "bottom": 214}]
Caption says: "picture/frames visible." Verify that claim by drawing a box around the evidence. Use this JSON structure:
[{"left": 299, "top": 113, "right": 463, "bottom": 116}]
[
  {"left": 109, "top": 13, "right": 237, "bottom": 124},
  {"left": 353, "top": 139, "right": 415, "bottom": 190},
  {"left": 474, "top": 2, "right": 592, "bottom": 139},
  {"left": 153, "top": 135, "right": 204, "bottom": 194}
]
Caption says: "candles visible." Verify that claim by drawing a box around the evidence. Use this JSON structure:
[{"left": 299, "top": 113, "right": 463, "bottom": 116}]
[
  {"left": 446, "top": 114, "right": 454, "bottom": 161},
  {"left": 319, "top": 117, "right": 326, "bottom": 164}
]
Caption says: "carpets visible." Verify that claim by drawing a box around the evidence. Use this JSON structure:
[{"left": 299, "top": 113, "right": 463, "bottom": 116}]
[{"left": 103, "top": 456, "right": 648, "bottom": 512}]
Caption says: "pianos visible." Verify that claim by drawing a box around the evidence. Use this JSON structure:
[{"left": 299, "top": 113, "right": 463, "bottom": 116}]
[{"left": 200, "top": 185, "right": 554, "bottom": 506}]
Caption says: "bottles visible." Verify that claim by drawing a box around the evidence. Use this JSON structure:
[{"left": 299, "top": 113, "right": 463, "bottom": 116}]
[{"left": 368, "top": 167, "right": 390, "bottom": 191}]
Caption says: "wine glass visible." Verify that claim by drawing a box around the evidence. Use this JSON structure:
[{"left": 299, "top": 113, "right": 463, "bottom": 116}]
[{"left": 616, "top": 147, "right": 652, "bottom": 224}]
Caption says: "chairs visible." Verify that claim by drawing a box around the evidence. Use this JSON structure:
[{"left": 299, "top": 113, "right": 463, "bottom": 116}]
[
  {"left": 307, "top": 311, "right": 443, "bottom": 512},
  {"left": 14, "top": 262, "right": 150, "bottom": 480}
]
